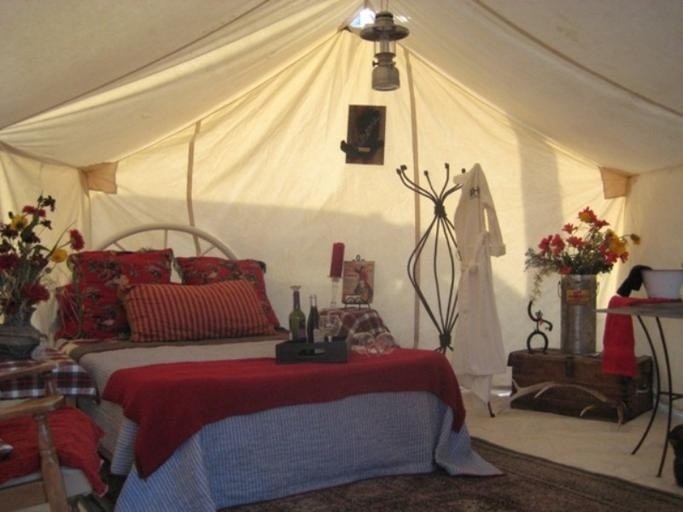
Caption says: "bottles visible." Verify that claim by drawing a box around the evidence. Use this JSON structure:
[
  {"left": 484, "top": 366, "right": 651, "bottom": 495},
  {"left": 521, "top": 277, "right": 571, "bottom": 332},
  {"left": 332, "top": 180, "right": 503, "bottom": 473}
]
[
  {"left": 304, "top": 293, "right": 324, "bottom": 344},
  {"left": 289, "top": 290, "right": 307, "bottom": 342}
]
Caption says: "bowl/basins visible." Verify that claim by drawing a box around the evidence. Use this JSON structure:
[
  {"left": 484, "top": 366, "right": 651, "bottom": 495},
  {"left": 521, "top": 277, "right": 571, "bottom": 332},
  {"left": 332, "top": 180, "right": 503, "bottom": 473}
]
[{"left": 640, "top": 268, "right": 682, "bottom": 302}]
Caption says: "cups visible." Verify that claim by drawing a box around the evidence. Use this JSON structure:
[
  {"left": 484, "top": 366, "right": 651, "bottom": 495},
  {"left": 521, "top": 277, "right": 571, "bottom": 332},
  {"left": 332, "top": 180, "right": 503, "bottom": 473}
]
[{"left": 349, "top": 331, "right": 396, "bottom": 356}]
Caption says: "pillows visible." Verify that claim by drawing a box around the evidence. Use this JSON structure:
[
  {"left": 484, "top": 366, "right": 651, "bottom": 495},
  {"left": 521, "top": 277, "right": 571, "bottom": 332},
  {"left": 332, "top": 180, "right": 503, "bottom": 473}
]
[
  {"left": 54, "top": 283, "right": 80, "bottom": 337},
  {"left": 123, "top": 278, "right": 278, "bottom": 342},
  {"left": 68, "top": 249, "right": 173, "bottom": 340},
  {"left": 172, "top": 256, "right": 288, "bottom": 335}
]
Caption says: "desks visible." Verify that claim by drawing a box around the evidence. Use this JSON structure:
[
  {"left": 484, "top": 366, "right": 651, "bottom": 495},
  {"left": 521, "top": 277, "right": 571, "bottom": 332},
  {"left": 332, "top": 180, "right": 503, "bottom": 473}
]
[
  {"left": 317, "top": 307, "right": 390, "bottom": 347},
  {"left": 597, "top": 300, "right": 683, "bottom": 476},
  {"left": 0, "top": 345, "right": 100, "bottom": 509}
]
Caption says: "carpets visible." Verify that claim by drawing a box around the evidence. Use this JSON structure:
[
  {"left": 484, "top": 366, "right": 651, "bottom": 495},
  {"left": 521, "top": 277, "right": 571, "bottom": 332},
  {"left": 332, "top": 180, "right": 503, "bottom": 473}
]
[{"left": 230, "top": 436, "right": 679, "bottom": 509}]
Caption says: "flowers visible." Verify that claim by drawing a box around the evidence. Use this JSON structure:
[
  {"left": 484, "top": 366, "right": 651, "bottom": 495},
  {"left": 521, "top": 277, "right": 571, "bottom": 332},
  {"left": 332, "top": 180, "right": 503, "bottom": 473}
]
[
  {"left": 0, "top": 188, "right": 84, "bottom": 313},
  {"left": 521, "top": 209, "right": 641, "bottom": 296}
]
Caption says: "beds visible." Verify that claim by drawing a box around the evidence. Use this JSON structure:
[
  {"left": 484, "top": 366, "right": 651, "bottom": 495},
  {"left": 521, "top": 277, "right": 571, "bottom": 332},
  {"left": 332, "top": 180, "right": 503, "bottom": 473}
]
[{"left": 52, "top": 223, "right": 503, "bottom": 510}]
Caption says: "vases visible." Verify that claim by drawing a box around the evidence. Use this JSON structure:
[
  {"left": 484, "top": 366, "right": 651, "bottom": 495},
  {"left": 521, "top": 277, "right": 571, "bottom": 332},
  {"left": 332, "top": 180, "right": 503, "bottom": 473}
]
[
  {"left": 560, "top": 275, "right": 596, "bottom": 353},
  {"left": 0, "top": 306, "right": 38, "bottom": 358}
]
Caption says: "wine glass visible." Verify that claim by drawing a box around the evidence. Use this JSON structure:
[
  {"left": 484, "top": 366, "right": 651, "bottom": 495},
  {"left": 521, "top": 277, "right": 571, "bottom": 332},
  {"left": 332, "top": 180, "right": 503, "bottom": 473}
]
[{"left": 318, "top": 307, "right": 341, "bottom": 342}]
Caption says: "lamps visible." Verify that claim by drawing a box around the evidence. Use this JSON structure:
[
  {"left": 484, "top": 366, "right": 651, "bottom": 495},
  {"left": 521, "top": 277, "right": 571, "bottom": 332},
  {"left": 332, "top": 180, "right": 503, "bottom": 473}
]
[{"left": 359, "top": 0, "right": 410, "bottom": 91}]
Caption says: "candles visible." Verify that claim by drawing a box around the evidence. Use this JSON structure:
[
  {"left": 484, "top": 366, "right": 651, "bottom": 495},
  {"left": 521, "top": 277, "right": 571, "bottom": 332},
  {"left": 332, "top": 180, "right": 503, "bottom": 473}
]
[{"left": 330, "top": 241, "right": 344, "bottom": 275}]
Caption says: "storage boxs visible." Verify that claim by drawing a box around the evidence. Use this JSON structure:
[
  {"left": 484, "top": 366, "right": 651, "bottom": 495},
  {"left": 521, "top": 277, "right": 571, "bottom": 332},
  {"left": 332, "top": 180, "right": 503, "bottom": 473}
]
[
  {"left": 272, "top": 335, "right": 354, "bottom": 364},
  {"left": 506, "top": 348, "right": 652, "bottom": 420}
]
